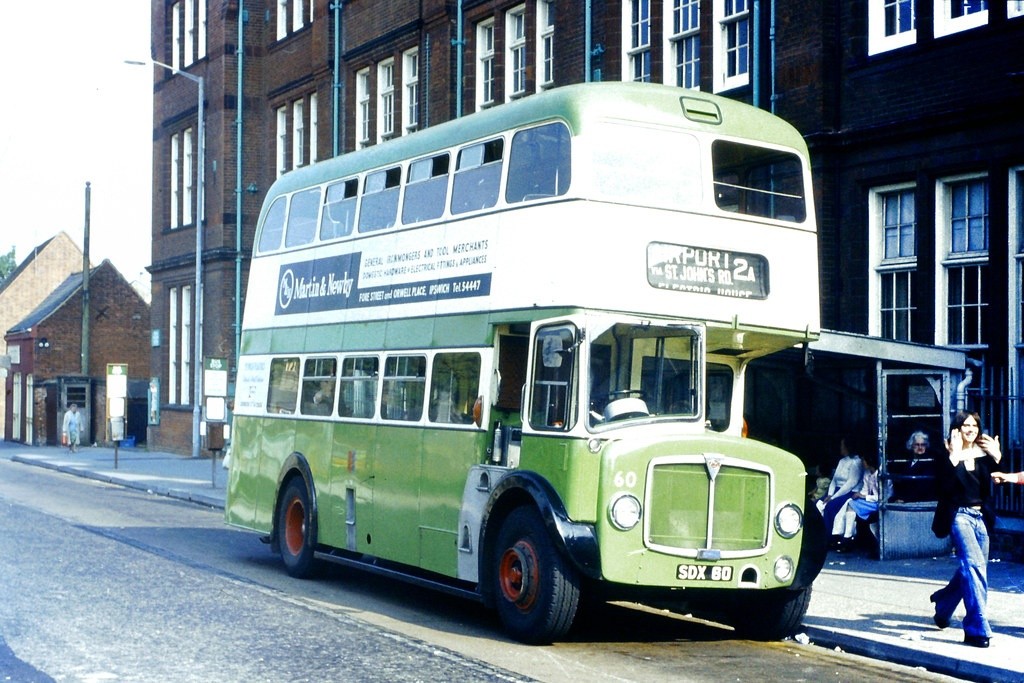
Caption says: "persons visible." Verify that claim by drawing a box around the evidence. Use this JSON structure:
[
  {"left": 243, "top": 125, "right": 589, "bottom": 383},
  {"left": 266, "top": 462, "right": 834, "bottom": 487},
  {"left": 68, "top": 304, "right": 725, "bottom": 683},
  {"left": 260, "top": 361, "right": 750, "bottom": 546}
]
[
  {"left": 808, "top": 461, "right": 831, "bottom": 502},
  {"left": 990, "top": 472, "right": 1024, "bottom": 484},
  {"left": 930, "top": 411, "right": 1001, "bottom": 646},
  {"left": 895, "top": 431, "right": 933, "bottom": 503},
  {"left": 832, "top": 451, "right": 894, "bottom": 551},
  {"left": 313, "top": 370, "right": 334, "bottom": 403},
  {"left": 817, "top": 433, "right": 865, "bottom": 537},
  {"left": 63, "top": 404, "right": 83, "bottom": 452}
]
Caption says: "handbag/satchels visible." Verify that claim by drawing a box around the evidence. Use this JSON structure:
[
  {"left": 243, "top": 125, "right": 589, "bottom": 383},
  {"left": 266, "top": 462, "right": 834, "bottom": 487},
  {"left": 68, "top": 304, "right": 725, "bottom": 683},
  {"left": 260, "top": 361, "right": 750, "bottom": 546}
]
[{"left": 62, "top": 432, "right": 67, "bottom": 444}]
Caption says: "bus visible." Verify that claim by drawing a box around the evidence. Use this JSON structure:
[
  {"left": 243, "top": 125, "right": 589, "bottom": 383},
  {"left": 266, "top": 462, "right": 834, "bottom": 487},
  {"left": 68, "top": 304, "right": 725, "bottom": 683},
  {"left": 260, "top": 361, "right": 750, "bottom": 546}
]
[{"left": 220, "top": 80, "right": 832, "bottom": 639}]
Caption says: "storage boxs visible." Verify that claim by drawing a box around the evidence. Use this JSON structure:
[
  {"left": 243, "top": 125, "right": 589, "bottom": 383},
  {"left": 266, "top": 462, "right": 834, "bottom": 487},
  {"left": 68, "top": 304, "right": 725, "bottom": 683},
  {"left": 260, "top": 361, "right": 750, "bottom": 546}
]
[{"left": 119, "top": 436, "right": 136, "bottom": 448}]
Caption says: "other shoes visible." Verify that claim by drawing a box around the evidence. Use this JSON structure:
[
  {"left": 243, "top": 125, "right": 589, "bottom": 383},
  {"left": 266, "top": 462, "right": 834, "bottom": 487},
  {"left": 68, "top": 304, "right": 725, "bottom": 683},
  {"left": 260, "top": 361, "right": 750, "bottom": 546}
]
[{"left": 834, "top": 538, "right": 854, "bottom": 550}]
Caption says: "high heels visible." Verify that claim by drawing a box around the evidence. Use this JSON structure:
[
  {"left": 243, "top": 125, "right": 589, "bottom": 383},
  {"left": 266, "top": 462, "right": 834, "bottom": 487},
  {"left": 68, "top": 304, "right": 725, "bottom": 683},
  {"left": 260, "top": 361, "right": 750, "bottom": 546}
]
[
  {"left": 930, "top": 592, "right": 949, "bottom": 628},
  {"left": 965, "top": 632, "right": 989, "bottom": 647}
]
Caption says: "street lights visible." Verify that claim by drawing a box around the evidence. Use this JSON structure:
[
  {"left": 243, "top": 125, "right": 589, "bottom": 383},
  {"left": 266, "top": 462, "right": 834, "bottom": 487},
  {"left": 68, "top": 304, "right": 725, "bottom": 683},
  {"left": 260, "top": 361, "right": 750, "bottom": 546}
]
[{"left": 123, "top": 56, "right": 203, "bottom": 460}]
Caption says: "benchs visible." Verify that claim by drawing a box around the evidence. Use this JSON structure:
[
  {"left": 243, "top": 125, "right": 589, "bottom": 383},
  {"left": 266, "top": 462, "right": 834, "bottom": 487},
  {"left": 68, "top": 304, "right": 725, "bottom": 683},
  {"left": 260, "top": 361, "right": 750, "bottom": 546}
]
[{"left": 332, "top": 193, "right": 557, "bottom": 240}]
